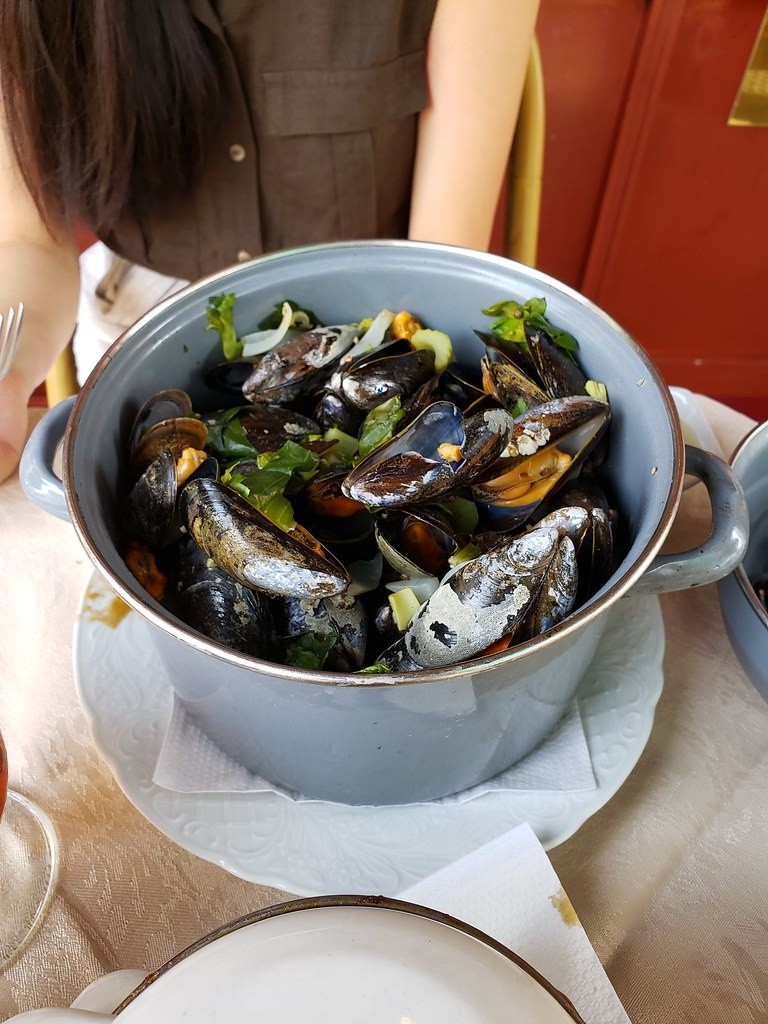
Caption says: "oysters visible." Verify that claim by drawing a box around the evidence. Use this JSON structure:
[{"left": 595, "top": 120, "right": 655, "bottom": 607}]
[{"left": 130, "top": 318, "right": 621, "bottom": 672}]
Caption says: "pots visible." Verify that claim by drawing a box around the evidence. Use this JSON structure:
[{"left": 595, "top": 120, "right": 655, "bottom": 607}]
[{"left": 21, "top": 238, "right": 752, "bottom": 809}]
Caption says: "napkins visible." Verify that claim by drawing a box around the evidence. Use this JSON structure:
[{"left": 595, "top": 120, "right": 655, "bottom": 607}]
[
  {"left": 153, "top": 672, "right": 598, "bottom": 808},
  {"left": 390, "top": 823, "right": 637, "bottom": 1024}
]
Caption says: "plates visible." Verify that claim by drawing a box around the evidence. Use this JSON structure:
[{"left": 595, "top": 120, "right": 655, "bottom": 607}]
[{"left": 72, "top": 564, "right": 670, "bottom": 898}]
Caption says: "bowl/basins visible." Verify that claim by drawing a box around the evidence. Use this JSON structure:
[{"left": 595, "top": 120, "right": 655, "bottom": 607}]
[
  {"left": 718, "top": 420, "right": 767, "bottom": 709},
  {"left": 0, "top": 892, "right": 584, "bottom": 1024}
]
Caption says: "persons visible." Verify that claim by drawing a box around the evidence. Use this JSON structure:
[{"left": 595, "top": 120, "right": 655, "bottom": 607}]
[{"left": 0, "top": 0, "right": 543, "bottom": 484}]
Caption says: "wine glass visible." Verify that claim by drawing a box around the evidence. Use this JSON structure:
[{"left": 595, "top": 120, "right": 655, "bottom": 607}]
[{"left": 0, "top": 729, "right": 63, "bottom": 975}]
